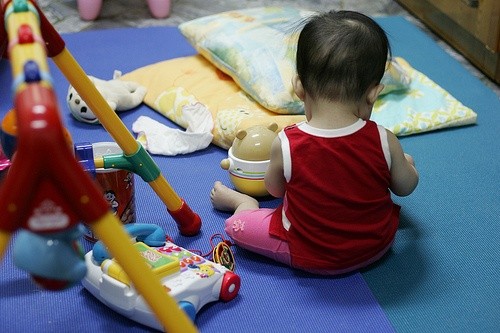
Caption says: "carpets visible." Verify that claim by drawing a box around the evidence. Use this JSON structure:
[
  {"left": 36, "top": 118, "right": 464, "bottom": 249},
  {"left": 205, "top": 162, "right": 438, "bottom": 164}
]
[{"left": 0, "top": 12, "right": 500, "bottom": 333}]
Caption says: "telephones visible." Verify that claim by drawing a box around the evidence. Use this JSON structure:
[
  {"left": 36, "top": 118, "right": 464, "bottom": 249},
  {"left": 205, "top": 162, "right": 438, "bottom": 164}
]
[{"left": 82, "top": 223, "right": 241, "bottom": 331}]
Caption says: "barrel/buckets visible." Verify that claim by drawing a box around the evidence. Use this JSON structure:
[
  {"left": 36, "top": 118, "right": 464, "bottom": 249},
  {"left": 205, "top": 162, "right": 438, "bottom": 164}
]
[{"left": 80, "top": 142, "right": 137, "bottom": 244}]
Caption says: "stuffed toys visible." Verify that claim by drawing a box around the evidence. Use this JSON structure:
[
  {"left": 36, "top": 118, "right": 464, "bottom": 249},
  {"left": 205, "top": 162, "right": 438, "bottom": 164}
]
[{"left": 67, "top": 75, "right": 146, "bottom": 124}]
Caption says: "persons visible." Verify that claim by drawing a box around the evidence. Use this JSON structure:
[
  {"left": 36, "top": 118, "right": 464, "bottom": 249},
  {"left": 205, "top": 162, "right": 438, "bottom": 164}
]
[{"left": 209, "top": 10, "right": 419, "bottom": 274}]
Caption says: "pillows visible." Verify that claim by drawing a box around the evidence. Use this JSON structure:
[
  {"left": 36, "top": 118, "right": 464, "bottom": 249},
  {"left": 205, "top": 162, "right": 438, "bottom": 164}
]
[
  {"left": 178, "top": 5, "right": 413, "bottom": 116},
  {"left": 116, "top": 49, "right": 478, "bottom": 148}
]
